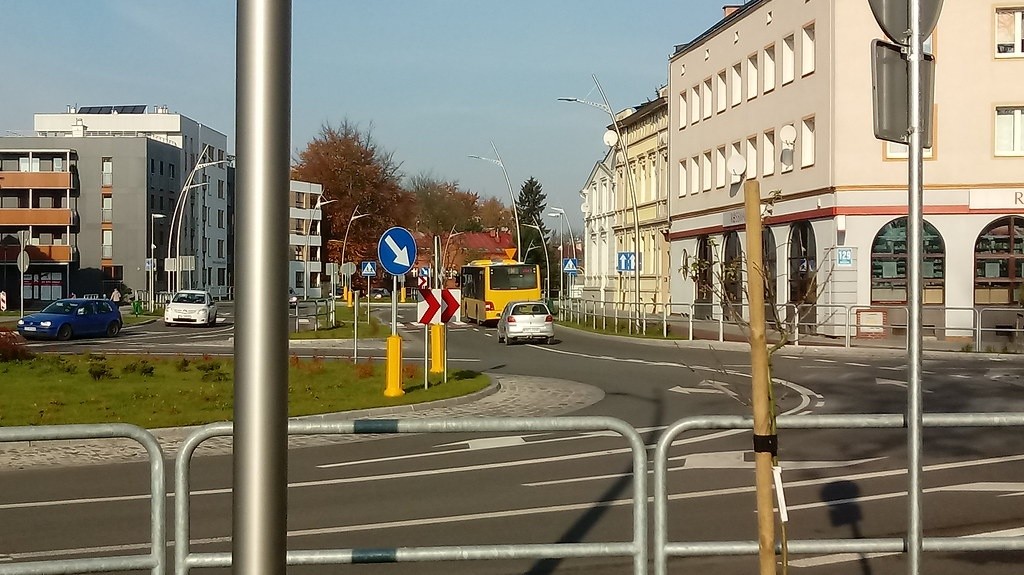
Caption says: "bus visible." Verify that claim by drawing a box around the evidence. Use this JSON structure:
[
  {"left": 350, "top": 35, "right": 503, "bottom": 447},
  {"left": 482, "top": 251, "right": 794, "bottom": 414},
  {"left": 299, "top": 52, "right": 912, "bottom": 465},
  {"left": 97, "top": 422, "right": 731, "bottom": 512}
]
[{"left": 460, "top": 260, "right": 543, "bottom": 326}]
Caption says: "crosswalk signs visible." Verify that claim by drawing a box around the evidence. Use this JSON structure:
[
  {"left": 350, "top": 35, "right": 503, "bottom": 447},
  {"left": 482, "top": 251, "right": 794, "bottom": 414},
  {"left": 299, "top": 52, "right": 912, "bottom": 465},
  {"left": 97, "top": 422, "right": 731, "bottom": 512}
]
[
  {"left": 562, "top": 258, "right": 578, "bottom": 275},
  {"left": 362, "top": 261, "right": 376, "bottom": 276}
]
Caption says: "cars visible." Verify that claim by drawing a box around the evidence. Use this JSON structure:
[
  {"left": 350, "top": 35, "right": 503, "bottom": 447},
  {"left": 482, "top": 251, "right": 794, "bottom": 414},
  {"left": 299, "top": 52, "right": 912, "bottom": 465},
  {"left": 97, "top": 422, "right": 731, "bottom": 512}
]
[
  {"left": 497, "top": 301, "right": 555, "bottom": 345},
  {"left": 164, "top": 290, "right": 219, "bottom": 327},
  {"left": 16, "top": 298, "right": 123, "bottom": 341}
]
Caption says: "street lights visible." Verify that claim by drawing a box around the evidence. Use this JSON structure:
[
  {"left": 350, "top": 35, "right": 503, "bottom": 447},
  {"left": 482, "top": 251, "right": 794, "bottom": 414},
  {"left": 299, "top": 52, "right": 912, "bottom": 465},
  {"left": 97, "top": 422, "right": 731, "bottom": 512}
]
[
  {"left": 173, "top": 143, "right": 230, "bottom": 301},
  {"left": 467, "top": 137, "right": 523, "bottom": 264},
  {"left": 521, "top": 213, "right": 550, "bottom": 301},
  {"left": 558, "top": 71, "right": 643, "bottom": 335},
  {"left": 546, "top": 206, "right": 577, "bottom": 260},
  {"left": 341, "top": 204, "right": 370, "bottom": 285},
  {"left": 303, "top": 187, "right": 339, "bottom": 301},
  {"left": 167, "top": 166, "right": 209, "bottom": 294}
]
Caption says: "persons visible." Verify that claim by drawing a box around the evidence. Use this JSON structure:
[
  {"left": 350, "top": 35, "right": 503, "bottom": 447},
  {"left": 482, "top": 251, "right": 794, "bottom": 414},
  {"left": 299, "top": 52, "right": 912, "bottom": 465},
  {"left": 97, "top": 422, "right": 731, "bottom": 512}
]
[{"left": 109, "top": 287, "right": 122, "bottom": 311}]
